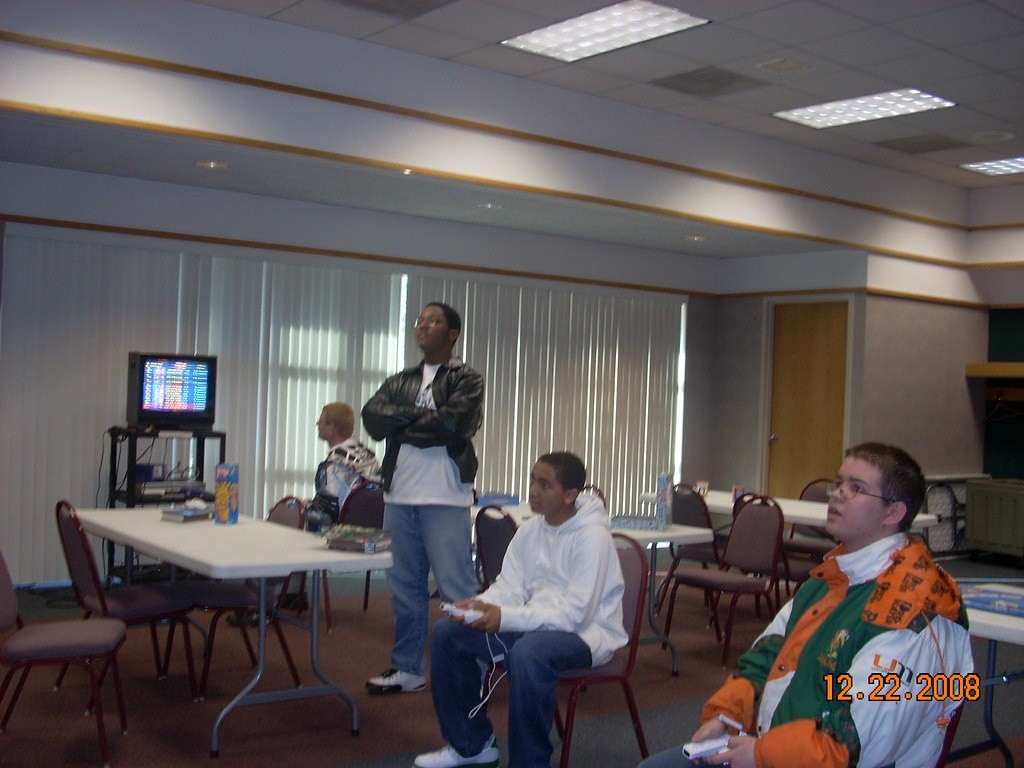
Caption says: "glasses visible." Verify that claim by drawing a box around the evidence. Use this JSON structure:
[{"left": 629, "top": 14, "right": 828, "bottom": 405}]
[
  {"left": 411, "top": 317, "right": 451, "bottom": 328},
  {"left": 824, "top": 481, "right": 896, "bottom": 503}
]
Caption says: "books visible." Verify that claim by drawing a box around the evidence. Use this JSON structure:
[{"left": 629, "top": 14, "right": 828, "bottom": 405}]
[
  {"left": 162, "top": 508, "right": 215, "bottom": 522},
  {"left": 328, "top": 533, "right": 392, "bottom": 552}
]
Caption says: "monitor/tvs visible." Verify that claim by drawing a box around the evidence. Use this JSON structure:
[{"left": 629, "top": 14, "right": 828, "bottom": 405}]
[{"left": 126, "top": 352, "right": 217, "bottom": 432}]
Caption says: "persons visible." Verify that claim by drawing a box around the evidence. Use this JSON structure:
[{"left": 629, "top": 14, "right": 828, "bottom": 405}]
[
  {"left": 412, "top": 452, "right": 629, "bottom": 768},
  {"left": 361, "top": 302, "right": 486, "bottom": 694},
  {"left": 225, "top": 402, "right": 379, "bottom": 627},
  {"left": 637, "top": 441, "right": 974, "bottom": 768}
]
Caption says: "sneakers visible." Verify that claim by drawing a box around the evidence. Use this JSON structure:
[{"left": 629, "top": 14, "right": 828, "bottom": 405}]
[
  {"left": 412, "top": 735, "right": 500, "bottom": 768},
  {"left": 365, "top": 669, "right": 426, "bottom": 693}
]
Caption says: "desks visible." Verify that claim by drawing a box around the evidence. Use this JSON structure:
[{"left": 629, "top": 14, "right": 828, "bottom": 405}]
[
  {"left": 693, "top": 490, "right": 942, "bottom": 589},
  {"left": 953, "top": 582, "right": 1024, "bottom": 768},
  {"left": 472, "top": 502, "right": 715, "bottom": 675},
  {"left": 76, "top": 506, "right": 393, "bottom": 756}
]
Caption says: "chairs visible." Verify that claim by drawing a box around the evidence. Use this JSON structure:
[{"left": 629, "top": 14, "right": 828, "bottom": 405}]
[{"left": 0, "top": 478, "right": 965, "bottom": 768}]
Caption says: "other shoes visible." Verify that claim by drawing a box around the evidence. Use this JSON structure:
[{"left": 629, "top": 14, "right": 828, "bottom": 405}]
[
  {"left": 225, "top": 609, "right": 274, "bottom": 626},
  {"left": 279, "top": 591, "right": 309, "bottom": 610}
]
